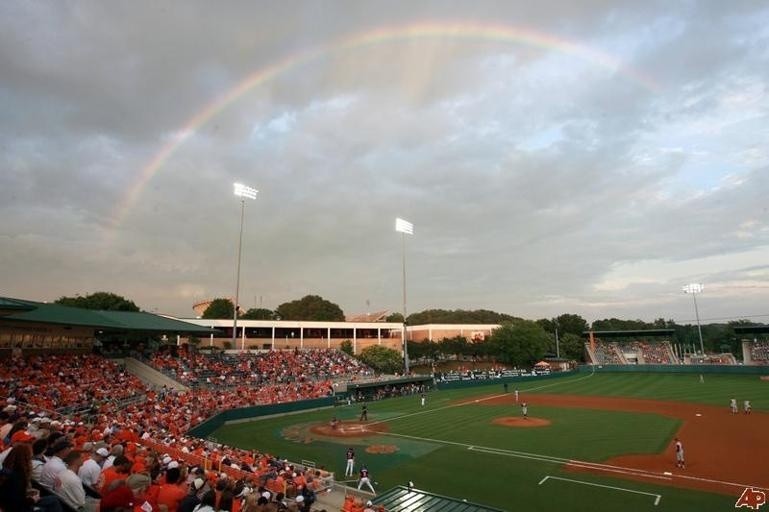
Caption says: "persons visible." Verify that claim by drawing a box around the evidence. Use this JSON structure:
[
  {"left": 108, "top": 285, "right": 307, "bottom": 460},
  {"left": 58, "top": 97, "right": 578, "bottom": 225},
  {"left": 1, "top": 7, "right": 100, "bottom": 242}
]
[
  {"left": 675, "top": 438, "right": 685, "bottom": 471},
  {"left": 1, "top": 340, "right": 527, "bottom": 512},
  {"left": 751, "top": 343, "right": 769, "bottom": 360},
  {"left": 730, "top": 398, "right": 738, "bottom": 415},
  {"left": 593, "top": 342, "right": 673, "bottom": 364},
  {"left": 744, "top": 400, "right": 751, "bottom": 415}
]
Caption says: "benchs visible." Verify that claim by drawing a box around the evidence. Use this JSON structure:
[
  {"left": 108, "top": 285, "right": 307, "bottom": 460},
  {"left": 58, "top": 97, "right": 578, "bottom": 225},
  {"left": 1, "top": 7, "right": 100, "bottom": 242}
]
[
  {"left": 0, "top": 334, "right": 400, "bottom": 511},
  {"left": 580, "top": 333, "right": 769, "bottom": 375}
]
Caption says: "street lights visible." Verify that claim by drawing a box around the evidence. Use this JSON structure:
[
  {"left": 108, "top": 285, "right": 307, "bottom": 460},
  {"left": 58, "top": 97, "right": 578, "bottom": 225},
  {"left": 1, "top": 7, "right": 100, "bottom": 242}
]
[
  {"left": 228, "top": 175, "right": 258, "bottom": 351},
  {"left": 395, "top": 214, "right": 416, "bottom": 371},
  {"left": 680, "top": 279, "right": 708, "bottom": 357}
]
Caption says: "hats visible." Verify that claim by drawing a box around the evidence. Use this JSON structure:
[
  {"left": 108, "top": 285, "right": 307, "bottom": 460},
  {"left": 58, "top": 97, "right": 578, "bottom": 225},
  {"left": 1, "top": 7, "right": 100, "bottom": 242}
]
[
  {"left": 3, "top": 405, "right": 17, "bottom": 411},
  {"left": 295, "top": 495, "right": 304, "bottom": 502},
  {"left": 126, "top": 472, "right": 151, "bottom": 489},
  {"left": 233, "top": 486, "right": 244, "bottom": 498},
  {"left": 48, "top": 431, "right": 74, "bottom": 452},
  {"left": 163, "top": 456, "right": 172, "bottom": 464},
  {"left": 96, "top": 448, "right": 108, "bottom": 457},
  {"left": 188, "top": 464, "right": 199, "bottom": 473},
  {"left": 31, "top": 417, "right": 75, "bottom": 429},
  {"left": 190, "top": 477, "right": 208, "bottom": 491},
  {"left": 168, "top": 461, "right": 179, "bottom": 469},
  {"left": 262, "top": 492, "right": 270, "bottom": 498}
]
